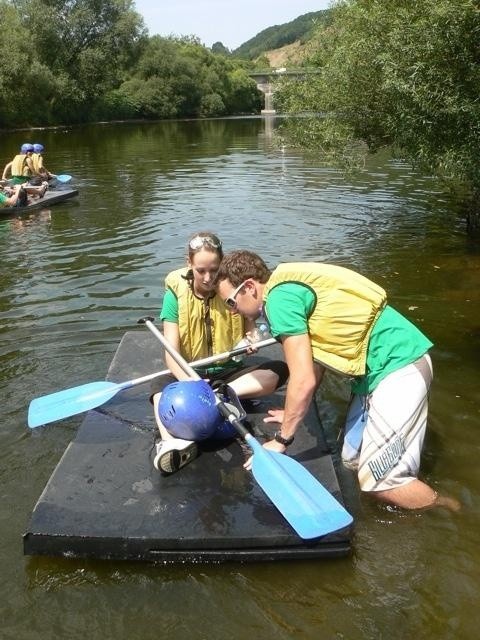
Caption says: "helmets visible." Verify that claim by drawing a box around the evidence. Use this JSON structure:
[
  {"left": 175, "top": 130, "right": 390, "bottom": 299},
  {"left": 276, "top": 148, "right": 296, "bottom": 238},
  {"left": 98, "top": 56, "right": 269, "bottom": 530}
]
[
  {"left": 21, "top": 143, "right": 44, "bottom": 154},
  {"left": 158, "top": 379, "right": 247, "bottom": 443}
]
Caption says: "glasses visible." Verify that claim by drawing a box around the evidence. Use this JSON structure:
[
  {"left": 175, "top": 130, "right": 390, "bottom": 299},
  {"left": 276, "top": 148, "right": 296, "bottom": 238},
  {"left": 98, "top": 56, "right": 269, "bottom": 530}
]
[
  {"left": 189, "top": 236, "right": 223, "bottom": 250},
  {"left": 224, "top": 277, "right": 253, "bottom": 309}
]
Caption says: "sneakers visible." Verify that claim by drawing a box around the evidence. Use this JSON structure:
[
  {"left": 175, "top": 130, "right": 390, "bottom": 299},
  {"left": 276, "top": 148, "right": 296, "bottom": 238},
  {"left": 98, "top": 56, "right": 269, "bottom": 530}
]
[{"left": 154, "top": 438, "right": 199, "bottom": 473}]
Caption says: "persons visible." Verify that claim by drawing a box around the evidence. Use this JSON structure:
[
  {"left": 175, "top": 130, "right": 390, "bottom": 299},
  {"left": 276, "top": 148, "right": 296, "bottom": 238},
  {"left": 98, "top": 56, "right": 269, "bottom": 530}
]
[
  {"left": 153, "top": 232, "right": 289, "bottom": 480},
  {"left": 211, "top": 248, "right": 461, "bottom": 523},
  {"left": 0, "top": 143, "right": 53, "bottom": 209}
]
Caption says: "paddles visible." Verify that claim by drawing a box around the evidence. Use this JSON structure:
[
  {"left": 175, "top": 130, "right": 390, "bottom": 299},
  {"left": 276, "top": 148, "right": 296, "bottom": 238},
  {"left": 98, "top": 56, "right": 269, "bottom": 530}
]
[
  {"left": 50, "top": 174, "right": 71, "bottom": 183},
  {"left": 27, "top": 336, "right": 280, "bottom": 429},
  {"left": 137, "top": 316, "right": 354, "bottom": 539}
]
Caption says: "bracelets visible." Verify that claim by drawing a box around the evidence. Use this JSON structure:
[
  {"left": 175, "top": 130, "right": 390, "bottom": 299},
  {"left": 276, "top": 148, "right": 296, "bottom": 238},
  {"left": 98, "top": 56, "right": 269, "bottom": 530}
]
[{"left": 275, "top": 432, "right": 295, "bottom": 447}]
